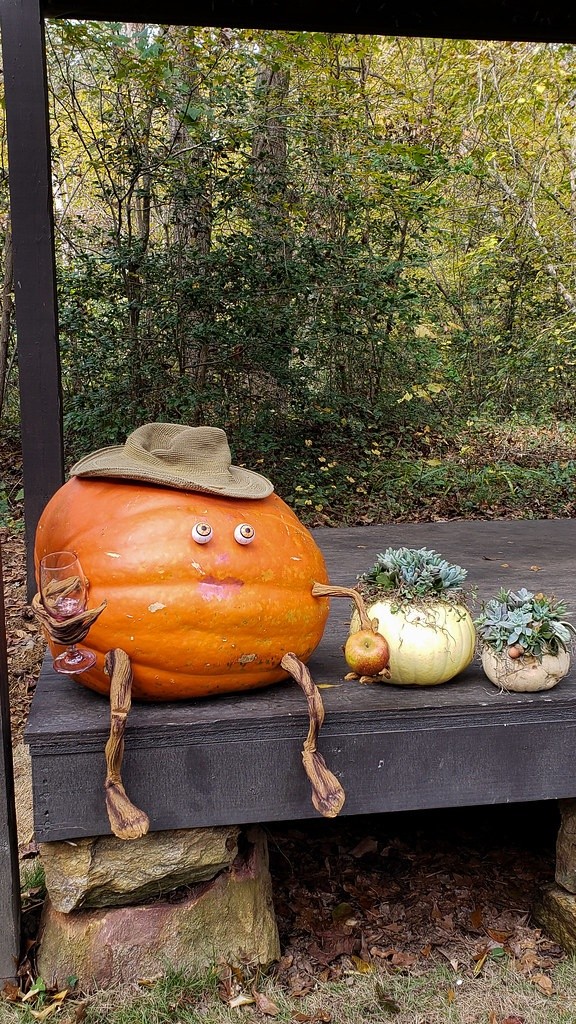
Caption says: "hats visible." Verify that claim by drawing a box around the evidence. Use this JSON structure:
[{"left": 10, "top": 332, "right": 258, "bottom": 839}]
[{"left": 69, "top": 422, "right": 275, "bottom": 501}]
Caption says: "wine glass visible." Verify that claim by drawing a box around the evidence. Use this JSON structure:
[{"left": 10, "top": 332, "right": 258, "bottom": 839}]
[{"left": 40, "top": 552, "right": 96, "bottom": 675}]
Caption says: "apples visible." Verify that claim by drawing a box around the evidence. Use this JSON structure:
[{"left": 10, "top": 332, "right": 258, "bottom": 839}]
[{"left": 344, "top": 629, "right": 389, "bottom": 676}]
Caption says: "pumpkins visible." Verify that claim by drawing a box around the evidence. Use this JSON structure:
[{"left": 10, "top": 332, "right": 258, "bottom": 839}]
[
  {"left": 33, "top": 474, "right": 329, "bottom": 705},
  {"left": 481, "top": 642, "right": 570, "bottom": 692},
  {"left": 349, "top": 599, "right": 476, "bottom": 686}
]
